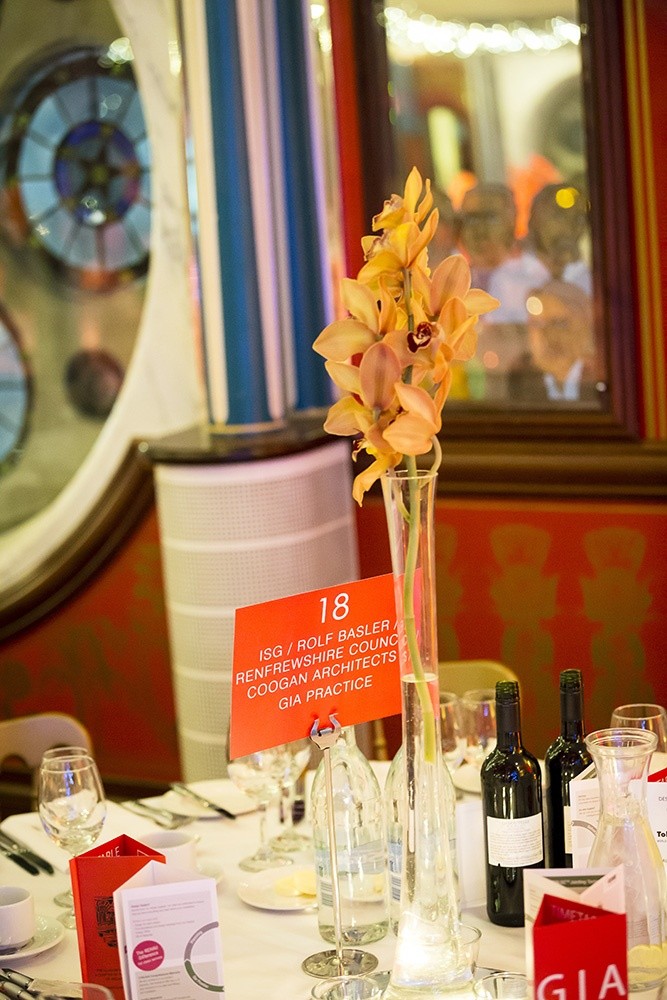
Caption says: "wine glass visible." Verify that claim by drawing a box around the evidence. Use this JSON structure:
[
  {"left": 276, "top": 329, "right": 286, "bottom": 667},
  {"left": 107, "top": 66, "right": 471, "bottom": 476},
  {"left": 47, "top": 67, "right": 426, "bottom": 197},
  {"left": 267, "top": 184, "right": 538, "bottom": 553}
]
[
  {"left": 224, "top": 710, "right": 315, "bottom": 873},
  {"left": 37, "top": 743, "right": 105, "bottom": 929},
  {"left": 460, "top": 685, "right": 498, "bottom": 798},
  {"left": 428, "top": 688, "right": 466, "bottom": 801}
]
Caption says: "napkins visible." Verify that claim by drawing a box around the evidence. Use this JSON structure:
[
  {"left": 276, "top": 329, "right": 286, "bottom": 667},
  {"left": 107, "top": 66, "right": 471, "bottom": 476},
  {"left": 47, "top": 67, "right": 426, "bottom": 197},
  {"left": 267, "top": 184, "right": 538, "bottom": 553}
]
[
  {"left": 3, "top": 800, "right": 160, "bottom": 875},
  {"left": 124, "top": 779, "right": 264, "bottom": 830}
]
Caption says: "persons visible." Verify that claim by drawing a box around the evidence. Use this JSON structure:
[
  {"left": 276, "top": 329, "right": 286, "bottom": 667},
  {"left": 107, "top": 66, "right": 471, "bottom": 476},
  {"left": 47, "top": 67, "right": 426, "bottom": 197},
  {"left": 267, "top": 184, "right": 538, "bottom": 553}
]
[{"left": 427, "top": 182, "right": 601, "bottom": 401}]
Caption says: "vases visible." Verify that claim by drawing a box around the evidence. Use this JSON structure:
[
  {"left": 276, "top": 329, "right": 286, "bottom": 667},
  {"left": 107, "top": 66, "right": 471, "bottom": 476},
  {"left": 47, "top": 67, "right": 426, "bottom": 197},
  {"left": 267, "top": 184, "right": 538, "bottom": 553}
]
[{"left": 380, "top": 468, "right": 477, "bottom": 995}]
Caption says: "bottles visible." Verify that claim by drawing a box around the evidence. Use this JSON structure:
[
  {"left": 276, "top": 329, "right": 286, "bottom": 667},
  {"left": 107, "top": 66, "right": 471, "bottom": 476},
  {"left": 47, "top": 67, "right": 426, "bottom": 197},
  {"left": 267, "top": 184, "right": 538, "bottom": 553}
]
[
  {"left": 315, "top": 715, "right": 389, "bottom": 947},
  {"left": 543, "top": 665, "right": 599, "bottom": 871},
  {"left": 573, "top": 728, "right": 666, "bottom": 1000},
  {"left": 475, "top": 678, "right": 549, "bottom": 924},
  {"left": 377, "top": 695, "right": 466, "bottom": 943}
]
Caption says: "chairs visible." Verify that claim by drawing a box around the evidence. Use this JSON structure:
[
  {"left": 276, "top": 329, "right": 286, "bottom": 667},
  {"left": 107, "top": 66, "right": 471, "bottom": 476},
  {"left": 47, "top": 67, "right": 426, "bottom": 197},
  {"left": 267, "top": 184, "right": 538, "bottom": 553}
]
[
  {"left": 0, "top": 710, "right": 94, "bottom": 831},
  {"left": 438, "top": 661, "right": 524, "bottom": 745}
]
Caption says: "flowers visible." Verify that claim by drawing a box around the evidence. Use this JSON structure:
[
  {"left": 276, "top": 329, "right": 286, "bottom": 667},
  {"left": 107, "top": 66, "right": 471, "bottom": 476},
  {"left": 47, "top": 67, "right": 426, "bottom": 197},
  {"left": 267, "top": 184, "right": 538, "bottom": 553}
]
[{"left": 311, "top": 161, "right": 504, "bottom": 766}]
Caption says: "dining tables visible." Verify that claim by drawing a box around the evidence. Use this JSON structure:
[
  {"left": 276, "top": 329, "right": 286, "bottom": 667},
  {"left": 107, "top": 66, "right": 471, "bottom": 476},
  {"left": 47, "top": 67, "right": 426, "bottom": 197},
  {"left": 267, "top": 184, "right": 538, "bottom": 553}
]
[{"left": 1, "top": 752, "right": 667, "bottom": 1000}]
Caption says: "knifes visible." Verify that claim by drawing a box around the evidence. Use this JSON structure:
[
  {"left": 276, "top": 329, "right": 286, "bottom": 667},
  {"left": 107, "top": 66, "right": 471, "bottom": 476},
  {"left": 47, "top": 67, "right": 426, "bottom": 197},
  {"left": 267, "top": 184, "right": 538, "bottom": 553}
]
[
  {"left": 170, "top": 781, "right": 236, "bottom": 822},
  {"left": 0, "top": 829, "right": 54, "bottom": 877}
]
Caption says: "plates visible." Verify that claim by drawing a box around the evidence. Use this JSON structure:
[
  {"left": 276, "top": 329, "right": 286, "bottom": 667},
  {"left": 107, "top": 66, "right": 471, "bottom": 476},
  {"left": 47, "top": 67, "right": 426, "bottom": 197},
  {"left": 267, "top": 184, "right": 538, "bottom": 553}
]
[
  {"left": 238, "top": 877, "right": 317, "bottom": 917},
  {"left": 451, "top": 758, "right": 548, "bottom": 796},
  {"left": 164, "top": 780, "right": 259, "bottom": 817},
  {"left": 0, "top": 914, "right": 65, "bottom": 962}
]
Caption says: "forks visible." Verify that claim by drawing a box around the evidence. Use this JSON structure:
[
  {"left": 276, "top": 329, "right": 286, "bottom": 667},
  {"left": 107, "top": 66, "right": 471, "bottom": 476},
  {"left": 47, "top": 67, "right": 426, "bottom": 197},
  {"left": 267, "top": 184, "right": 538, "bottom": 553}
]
[{"left": 116, "top": 799, "right": 196, "bottom": 831}]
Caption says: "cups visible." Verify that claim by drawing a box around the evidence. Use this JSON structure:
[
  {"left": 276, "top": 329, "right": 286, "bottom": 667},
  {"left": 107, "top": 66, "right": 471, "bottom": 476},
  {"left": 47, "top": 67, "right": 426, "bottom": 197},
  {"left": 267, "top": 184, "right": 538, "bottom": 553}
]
[
  {"left": 445, "top": 920, "right": 482, "bottom": 981},
  {"left": 607, "top": 704, "right": 667, "bottom": 787},
  {"left": 309, "top": 975, "right": 381, "bottom": 1000},
  {"left": 470, "top": 970, "right": 528, "bottom": 1000},
  {"left": 0, "top": 886, "right": 36, "bottom": 949}
]
